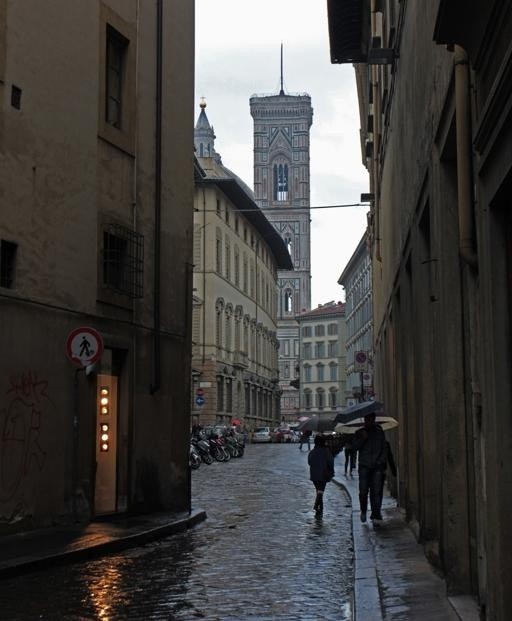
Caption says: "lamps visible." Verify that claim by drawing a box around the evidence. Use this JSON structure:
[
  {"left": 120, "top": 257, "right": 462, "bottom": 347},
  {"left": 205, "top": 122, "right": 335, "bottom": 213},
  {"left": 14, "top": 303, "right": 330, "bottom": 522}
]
[
  {"left": 359, "top": 192, "right": 375, "bottom": 205},
  {"left": 365, "top": 46, "right": 400, "bottom": 70}
]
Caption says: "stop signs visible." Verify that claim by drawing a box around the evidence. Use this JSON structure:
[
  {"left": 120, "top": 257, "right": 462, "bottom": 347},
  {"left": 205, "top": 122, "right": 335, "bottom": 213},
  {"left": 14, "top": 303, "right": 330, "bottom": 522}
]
[{"left": 196, "top": 389, "right": 204, "bottom": 396}]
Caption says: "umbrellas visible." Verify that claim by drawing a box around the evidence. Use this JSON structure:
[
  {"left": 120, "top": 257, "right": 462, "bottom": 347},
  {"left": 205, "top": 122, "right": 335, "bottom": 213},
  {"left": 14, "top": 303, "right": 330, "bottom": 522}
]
[
  {"left": 332, "top": 414, "right": 400, "bottom": 434},
  {"left": 333, "top": 399, "right": 383, "bottom": 426},
  {"left": 297, "top": 416, "right": 310, "bottom": 421},
  {"left": 293, "top": 415, "right": 335, "bottom": 435}
]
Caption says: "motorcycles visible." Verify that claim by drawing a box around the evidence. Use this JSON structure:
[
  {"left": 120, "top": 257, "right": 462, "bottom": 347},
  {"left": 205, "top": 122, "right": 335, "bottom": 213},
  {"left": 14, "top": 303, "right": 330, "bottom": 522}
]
[{"left": 190, "top": 424, "right": 244, "bottom": 469}]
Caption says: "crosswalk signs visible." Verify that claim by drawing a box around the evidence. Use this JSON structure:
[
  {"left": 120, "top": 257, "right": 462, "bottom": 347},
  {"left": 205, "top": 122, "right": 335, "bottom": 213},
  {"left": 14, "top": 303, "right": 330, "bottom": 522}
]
[{"left": 68, "top": 326, "right": 103, "bottom": 368}]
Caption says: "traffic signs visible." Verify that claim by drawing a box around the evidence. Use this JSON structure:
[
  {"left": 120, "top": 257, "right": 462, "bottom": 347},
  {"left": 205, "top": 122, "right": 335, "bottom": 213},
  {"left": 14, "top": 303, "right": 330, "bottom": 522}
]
[{"left": 354, "top": 351, "right": 369, "bottom": 373}]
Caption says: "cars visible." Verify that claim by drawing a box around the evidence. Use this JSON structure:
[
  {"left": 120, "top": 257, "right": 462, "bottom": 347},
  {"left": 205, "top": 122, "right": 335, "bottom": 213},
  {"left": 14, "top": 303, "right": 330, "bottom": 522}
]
[{"left": 251, "top": 424, "right": 299, "bottom": 443}]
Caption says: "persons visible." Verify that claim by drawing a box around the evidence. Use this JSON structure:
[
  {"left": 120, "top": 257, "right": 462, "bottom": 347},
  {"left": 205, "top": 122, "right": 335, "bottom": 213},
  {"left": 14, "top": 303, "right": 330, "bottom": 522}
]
[
  {"left": 343, "top": 447, "right": 356, "bottom": 480},
  {"left": 231, "top": 420, "right": 249, "bottom": 448},
  {"left": 298, "top": 430, "right": 313, "bottom": 450},
  {"left": 374, "top": 423, "right": 398, "bottom": 521},
  {"left": 308, "top": 435, "right": 336, "bottom": 513},
  {"left": 351, "top": 412, "right": 388, "bottom": 524}
]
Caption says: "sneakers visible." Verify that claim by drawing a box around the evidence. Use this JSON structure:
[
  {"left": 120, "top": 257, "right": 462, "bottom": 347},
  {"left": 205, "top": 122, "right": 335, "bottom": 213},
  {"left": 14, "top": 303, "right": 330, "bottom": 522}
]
[{"left": 360, "top": 510, "right": 367, "bottom": 523}]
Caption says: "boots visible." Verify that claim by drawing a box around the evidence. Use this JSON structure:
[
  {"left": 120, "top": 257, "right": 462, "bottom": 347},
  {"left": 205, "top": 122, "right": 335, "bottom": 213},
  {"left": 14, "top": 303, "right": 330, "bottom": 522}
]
[{"left": 313, "top": 493, "right": 324, "bottom": 514}]
